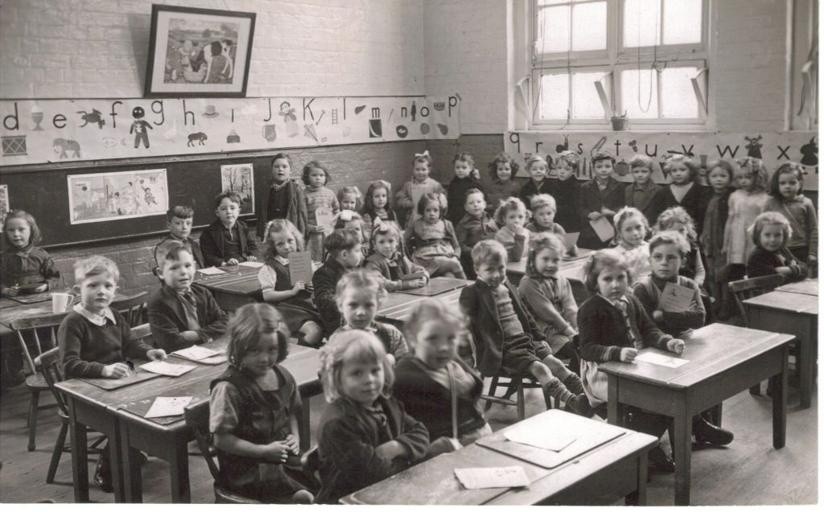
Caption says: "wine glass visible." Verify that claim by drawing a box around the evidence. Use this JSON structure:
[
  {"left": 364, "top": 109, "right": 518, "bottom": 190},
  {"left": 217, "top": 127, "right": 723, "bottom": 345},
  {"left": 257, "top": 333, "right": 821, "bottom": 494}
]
[{"left": 29, "top": 111, "right": 44, "bottom": 132}]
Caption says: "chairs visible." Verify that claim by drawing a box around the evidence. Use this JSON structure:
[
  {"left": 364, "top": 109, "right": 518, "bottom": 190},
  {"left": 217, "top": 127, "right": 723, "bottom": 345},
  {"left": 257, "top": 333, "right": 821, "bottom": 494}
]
[{"left": 1, "top": 213, "right": 800, "bottom": 504}]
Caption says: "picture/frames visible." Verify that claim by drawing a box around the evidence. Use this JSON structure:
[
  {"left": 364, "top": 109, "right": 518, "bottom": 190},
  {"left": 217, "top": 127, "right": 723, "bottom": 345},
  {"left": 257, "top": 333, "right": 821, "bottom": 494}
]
[
  {"left": 66, "top": 168, "right": 169, "bottom": 225},
  {"left": 221, "top": 162, "right": 256, "bottom": 216},
  {"left": 143, "top": 3, "right": 256, "bottom": 98}
]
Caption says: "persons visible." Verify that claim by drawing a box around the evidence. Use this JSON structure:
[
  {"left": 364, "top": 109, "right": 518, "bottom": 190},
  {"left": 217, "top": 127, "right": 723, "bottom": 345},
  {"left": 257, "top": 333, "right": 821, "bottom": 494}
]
[
  {"left": 57, "top": 255, "right": 168, "bottom": 492},
  {"left": 1, "top": 210, "right": 60, "bottom": 385},
  {"left": 145, "top": 151, "right": 818, "bottom": 505}
]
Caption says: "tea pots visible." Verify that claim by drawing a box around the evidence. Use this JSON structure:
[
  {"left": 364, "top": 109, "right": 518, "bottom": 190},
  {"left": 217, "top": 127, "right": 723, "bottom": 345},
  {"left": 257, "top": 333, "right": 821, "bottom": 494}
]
[{"left": 260, "top": 123, "right": 276, "bottom": 143}]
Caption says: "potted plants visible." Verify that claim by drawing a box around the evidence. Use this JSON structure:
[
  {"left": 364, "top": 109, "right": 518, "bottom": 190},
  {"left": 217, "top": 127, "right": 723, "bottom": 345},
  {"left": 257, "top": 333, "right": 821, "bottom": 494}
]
[{"left": 610, "top": 115, "right": 626, "bottom": 130}]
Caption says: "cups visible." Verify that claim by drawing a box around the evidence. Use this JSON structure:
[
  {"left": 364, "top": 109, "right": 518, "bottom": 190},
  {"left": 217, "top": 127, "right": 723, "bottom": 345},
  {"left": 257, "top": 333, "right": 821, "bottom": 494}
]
[
  {"left": 51, "top": 290, "right": 75, "bottom": 314},
  {"left": 368, "top": 120, "right": 382, "bottom": 139}
]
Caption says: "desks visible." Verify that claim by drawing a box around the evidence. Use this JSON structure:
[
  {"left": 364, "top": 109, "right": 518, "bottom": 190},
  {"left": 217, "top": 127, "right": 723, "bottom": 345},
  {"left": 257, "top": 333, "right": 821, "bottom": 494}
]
[{"left": 740, "top": 277, "right": 817, "bottom": 409}]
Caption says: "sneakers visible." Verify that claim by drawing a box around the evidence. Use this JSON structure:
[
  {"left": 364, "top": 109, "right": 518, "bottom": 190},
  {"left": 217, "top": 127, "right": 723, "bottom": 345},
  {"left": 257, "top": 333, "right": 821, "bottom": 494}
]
[
  {"left": 94, "top": 464, "right": 113, "bottom": 492},
  {"left": 695, "top": 418, "right": 733, "bottom": 445}
]
